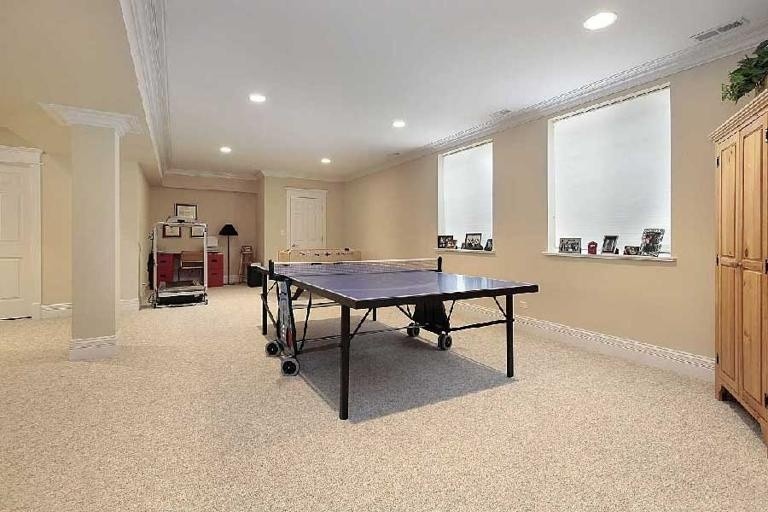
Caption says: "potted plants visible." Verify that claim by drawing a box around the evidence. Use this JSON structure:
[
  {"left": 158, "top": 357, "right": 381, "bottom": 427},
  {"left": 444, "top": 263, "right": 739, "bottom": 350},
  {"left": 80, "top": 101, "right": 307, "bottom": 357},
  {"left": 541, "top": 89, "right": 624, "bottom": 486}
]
[{"left": 720, "top": 39, "right": 768, "bottom": 104}]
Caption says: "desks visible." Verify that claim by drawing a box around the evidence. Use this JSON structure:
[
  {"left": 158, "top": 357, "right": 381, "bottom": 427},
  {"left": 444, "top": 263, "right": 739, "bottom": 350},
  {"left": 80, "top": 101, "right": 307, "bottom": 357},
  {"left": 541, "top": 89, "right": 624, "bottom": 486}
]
[
  {"left": 249, "top": 257, "right": 539, "bottom": 420},
  {"left": 156, "top": 251, "right": 224, "bottom": 288}
]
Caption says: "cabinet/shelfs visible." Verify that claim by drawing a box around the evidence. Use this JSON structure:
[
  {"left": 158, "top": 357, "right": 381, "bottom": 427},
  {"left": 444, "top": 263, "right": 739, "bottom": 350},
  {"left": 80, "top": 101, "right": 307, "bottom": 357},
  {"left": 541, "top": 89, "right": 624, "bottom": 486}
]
[{"left": 707, "top": 89, "right": 768, "bottom": 446}]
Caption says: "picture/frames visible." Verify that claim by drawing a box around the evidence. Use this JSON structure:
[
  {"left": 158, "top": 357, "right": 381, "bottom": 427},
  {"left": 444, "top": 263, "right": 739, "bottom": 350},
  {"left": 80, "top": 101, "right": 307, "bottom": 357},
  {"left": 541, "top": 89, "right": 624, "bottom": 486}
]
[
  {"left": 438, "top": 233, "right": 492, "bottom": 249},
  {"left": 163, "top": 202, "right": 205, "bottom": 238},
  {"left": 559, "top": 228, "right": 665, "bottom": 257}
]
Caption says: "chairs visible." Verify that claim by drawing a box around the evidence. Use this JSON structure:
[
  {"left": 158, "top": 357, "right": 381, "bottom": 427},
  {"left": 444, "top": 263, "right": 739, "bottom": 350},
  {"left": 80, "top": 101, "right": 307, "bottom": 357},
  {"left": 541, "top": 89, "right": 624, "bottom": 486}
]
[{"left": 177, "top": 250, "right": 204, "bottom": 286}]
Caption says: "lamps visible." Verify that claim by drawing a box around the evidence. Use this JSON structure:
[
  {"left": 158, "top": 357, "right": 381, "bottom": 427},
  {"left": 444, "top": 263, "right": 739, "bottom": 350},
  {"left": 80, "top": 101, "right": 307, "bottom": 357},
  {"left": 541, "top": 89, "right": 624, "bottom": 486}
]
[{"left": 219, "top": 224, "right": 238, "bottom": 285}]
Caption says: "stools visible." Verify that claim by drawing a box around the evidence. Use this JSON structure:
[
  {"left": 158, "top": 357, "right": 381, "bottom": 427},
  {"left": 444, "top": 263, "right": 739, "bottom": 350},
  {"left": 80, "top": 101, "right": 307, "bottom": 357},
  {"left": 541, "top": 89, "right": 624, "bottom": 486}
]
[{"left": 239, "top": 252, "right": 256, "bottom": 284}]
